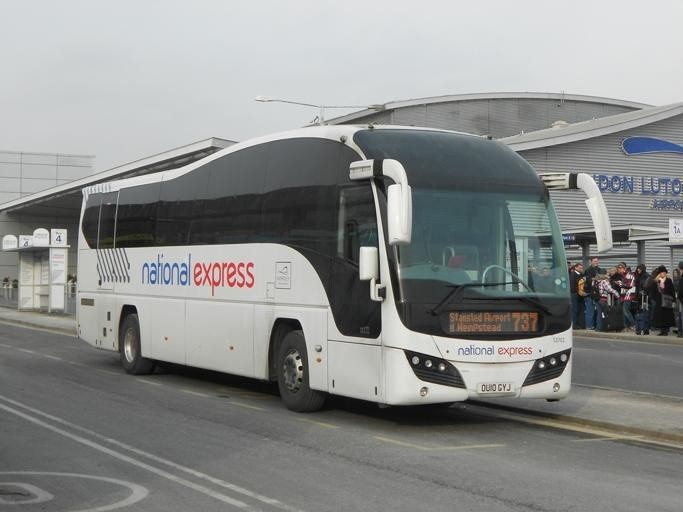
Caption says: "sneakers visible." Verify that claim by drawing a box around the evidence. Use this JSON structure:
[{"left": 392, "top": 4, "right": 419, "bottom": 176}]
[
  {"left": 573, "top": 324, "right": 603, "bottom": 332},
  {"left": 624, "top": 326, "right": 635, "bottom": 332}
]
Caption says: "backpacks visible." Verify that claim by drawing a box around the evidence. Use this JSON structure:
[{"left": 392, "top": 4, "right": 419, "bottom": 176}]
[
  {"left": 590, "top": 284, "right": 600, "bottom": 301},
  {"left": 577, "top": 276, "right": 591, "bottom": 296},
  {"left": 641, "top": 274, "right": 653, "bottom": 291}
]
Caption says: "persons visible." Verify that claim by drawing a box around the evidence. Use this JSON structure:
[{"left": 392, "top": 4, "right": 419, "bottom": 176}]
[
  {"left": 68, "top": 273, "right": 76, "bottom": 282},
  {"left": 527, "top": 257, "right": 683, "bottom": 339},
  {"left": 2, "top": 275, "right": 9, "bottom": 287}
]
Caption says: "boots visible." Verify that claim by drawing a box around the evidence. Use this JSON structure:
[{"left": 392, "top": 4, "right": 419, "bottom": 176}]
[{"left": 657, "top": 328, "right": 668, "bottom": 336}]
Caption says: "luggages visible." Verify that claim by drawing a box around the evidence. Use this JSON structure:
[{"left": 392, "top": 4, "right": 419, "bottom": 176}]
[
  {"left": 635, "top": 310, "right": 649, "bottom": 335},
  {"left": 604, "top": 305, "right": 623, "bottom": 332}
]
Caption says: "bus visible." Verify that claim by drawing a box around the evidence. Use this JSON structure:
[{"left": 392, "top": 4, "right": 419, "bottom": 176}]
[{"left": 73, "top": 124, "right": 612, "bottom": 414}]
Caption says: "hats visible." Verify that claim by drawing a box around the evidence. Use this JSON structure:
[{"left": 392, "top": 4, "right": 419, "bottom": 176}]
[{"left": 657, "top": 265, "right": 667, "bottom": 274}]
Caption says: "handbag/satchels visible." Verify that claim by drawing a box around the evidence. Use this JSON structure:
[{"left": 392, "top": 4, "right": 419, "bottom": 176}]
[{"left": 661, "top": 294, "right": 674, "bottom": 308}]
